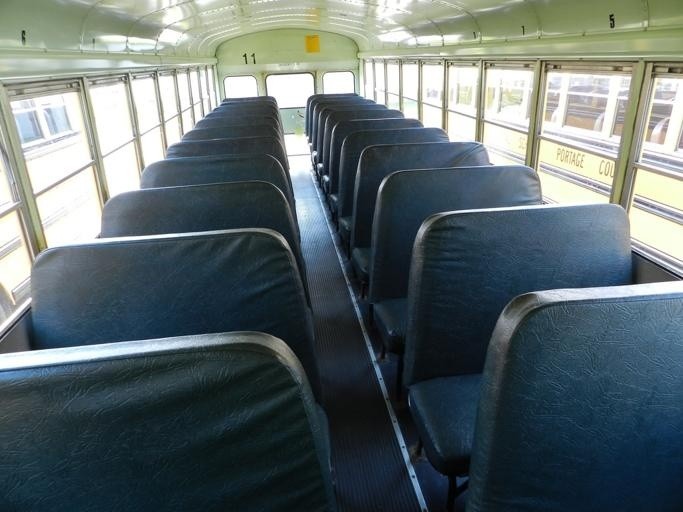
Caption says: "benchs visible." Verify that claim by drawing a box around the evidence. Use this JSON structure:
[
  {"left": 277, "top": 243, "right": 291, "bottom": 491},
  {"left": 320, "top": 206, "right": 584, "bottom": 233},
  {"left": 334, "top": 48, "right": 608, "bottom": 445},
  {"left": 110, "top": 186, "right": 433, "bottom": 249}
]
[
  {"left": 461, "top": 280, "right": 683, "bottom": 512},
  {"left": 303, "top": 92, "right": 451, "bottom": 245},
  {"left": 137, "top": 92, "right": 302, "bottom": 244},
  {"left": 99, "top": 180, "right": 316, "bottom": 346},
  {"left": 366, "top": 164, "right": 541, "bottom": 367},
  {"left": 347, "top": 141, "right": 492, "bottom": 300},
  {"left": 397, "top": 201, "right": 634, "bottom": 512},
  {"left": 0, "top": 332, "right": 336, "bottom": 512},
  {"left": 29, "top": 227, "right": 334, "bottom": 472}
]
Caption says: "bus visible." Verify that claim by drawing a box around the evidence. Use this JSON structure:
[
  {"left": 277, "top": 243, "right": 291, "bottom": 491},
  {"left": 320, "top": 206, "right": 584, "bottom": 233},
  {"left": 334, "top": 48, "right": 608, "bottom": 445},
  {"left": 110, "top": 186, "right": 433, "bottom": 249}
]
[
  {"left": 0, "top": 92, "right": 89, "bottom": 209},
  {"left": 421, "top": 73, "right": 683, "bottom": 260}
]
